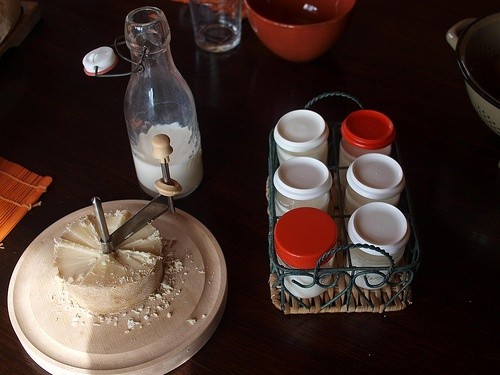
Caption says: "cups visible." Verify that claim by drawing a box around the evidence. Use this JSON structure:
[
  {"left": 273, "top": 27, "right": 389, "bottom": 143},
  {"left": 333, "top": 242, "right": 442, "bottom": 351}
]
[{"left": 190, "top": 0, "right": 242, "bottom": 52}]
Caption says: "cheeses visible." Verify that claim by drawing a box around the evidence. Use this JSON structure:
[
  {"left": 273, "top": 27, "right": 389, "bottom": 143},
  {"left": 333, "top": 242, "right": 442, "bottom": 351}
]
[{"left": 52, "top": 209, "right": 164, "bottom": 316}]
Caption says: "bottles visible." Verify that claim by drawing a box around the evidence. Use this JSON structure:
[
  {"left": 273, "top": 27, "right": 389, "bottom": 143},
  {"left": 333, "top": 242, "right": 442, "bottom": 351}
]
[
  {"left": 345, "top": 202, "right": 410, "bottom": 289},
  {"left": 124, "top": 6, "right": 203, "bottom": 200},
  {"left": 273, "top": 157, "right": 332, "bottom": 220},
  {"left": 339, "top": 110, "right": 396, "bottom": 192},
  {"left": 274, "top": 110, "right": 329, "bottom": 165},
  {"left": 274, "top": 208, "right": 339, "bottom": 298},
  {"left": 344, "top": 152, "right": 405, "bottom": 232}
]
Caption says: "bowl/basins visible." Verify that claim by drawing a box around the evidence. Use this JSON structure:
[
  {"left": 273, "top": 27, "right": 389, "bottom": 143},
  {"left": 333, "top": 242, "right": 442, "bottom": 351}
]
[
  {"left": 244, "top": 0, "right": 355, "bottom": 62},
  {"left": 446, "top": 12, "right": 500, "bottom": 134}
]
